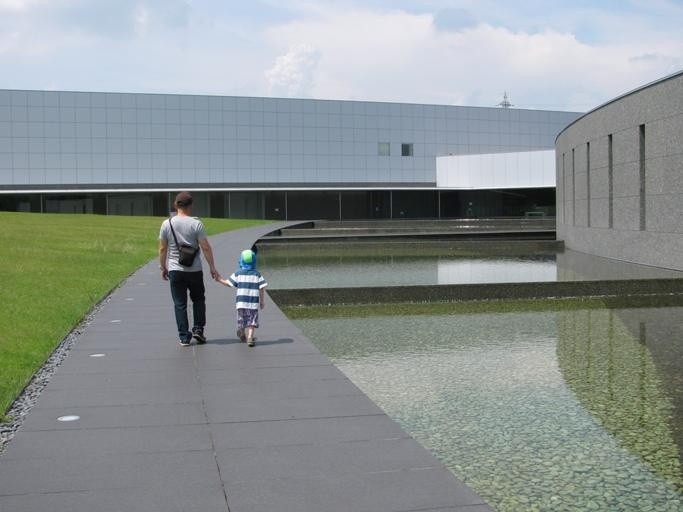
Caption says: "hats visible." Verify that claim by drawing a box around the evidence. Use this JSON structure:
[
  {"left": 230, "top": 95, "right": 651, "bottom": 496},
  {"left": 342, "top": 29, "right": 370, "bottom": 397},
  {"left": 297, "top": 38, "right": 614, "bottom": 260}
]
[
  {"left": 175, "top": 191, "right": 192, "bottom": 203},
  {"left": 239, "top": 249, "right": 256, "bottom": 270}
]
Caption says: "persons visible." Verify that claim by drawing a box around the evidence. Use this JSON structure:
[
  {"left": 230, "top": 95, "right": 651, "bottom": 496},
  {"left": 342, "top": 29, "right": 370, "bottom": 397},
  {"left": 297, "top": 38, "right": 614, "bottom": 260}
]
[
  {"left": 213, "top": 249, "right": 268, "bottom": 346},
  {"left": 158, "top": 191, "right": 218, "bottom": 347}
]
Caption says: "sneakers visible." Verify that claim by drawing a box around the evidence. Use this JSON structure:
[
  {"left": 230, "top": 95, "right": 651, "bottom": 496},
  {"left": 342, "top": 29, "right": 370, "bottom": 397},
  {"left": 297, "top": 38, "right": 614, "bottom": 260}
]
[
  {"left": 237, "top": 330, "right": 245, "bottom": 341},
  {"left": 179, "top": 340, "right": 190, "bottom": 346},
  {"left": 248, "top": 341, "right": 255, "bottom": 347},
  {"left": 192, "top": 330, "right": 206, "bottom": 343}
]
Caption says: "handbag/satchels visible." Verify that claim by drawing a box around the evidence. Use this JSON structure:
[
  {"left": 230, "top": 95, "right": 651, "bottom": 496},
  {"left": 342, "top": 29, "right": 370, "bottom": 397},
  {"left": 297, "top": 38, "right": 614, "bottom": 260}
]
[{"left": 178, "top": 245, "right": 197, "bottom": 267}]
